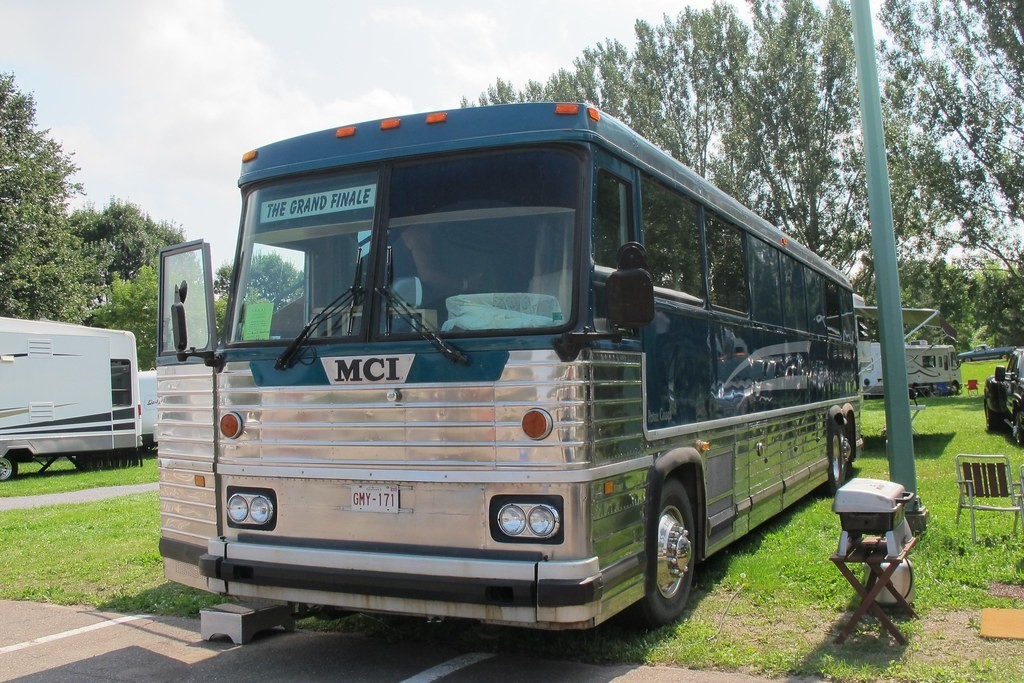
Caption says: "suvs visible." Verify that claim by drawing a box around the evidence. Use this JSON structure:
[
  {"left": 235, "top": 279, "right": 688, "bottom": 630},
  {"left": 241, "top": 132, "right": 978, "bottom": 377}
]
[{"left": 983, "top": 345, "right": 1024, "bottom": 446}]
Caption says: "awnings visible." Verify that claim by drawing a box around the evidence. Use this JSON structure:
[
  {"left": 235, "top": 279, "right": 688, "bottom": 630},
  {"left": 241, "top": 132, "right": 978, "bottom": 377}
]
[{"left": 853, "top": 305, "right": 957, "bottom": 391}]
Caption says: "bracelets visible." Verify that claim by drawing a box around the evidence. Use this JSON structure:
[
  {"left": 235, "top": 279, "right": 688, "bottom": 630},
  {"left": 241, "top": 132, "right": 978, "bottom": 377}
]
[{"left": 464, "top": 280, "right": 468, "bottom": 289}]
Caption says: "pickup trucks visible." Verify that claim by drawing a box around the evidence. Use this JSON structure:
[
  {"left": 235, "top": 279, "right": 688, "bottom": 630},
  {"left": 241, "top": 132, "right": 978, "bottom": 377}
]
[{"left": 957, "top": 344, "right": 1017, "bottom": 363}]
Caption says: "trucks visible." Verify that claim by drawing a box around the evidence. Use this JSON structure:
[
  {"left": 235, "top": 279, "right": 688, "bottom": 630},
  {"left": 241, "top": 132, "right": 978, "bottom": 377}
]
[{"left": 0, "top": 318, "right": 144, "bottom": 483}]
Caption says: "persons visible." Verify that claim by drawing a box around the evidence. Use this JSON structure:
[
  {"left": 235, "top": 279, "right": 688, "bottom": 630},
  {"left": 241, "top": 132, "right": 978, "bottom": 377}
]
[{"left": 389, "top": 227, "right": 476, "bottom": 293}]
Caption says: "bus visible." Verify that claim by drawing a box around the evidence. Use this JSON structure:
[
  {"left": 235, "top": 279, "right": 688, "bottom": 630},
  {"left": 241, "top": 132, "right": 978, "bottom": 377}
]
[
  {"left": 154, "top": 101, "right": 863, "bottom": 632},
  {"left": 906, "top": 345, "right": 966, "bottom": 398}
]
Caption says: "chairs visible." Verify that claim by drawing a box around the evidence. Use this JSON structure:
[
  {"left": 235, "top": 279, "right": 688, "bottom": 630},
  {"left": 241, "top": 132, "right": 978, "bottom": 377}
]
[
  {"left": 965, "top": 379, "right": 980, "bottom": 395},
  {"left": 953, "top": 453, "right": 1024, "bottom": 544},
  {"left": 936, "top": 381, "right": 951, "bottom": 397}
]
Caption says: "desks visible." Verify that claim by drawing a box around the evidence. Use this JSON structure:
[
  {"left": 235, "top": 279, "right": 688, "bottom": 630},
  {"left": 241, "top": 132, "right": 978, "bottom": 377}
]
[
  {"left": 916, "top": 386, "right": 935, "bottom": 397},
  {"left": 910, "top": 404, "right": 927, "bottom": 437},
  {"left": 829, "top": 534, "right": 918, "bottom": 646}
]
